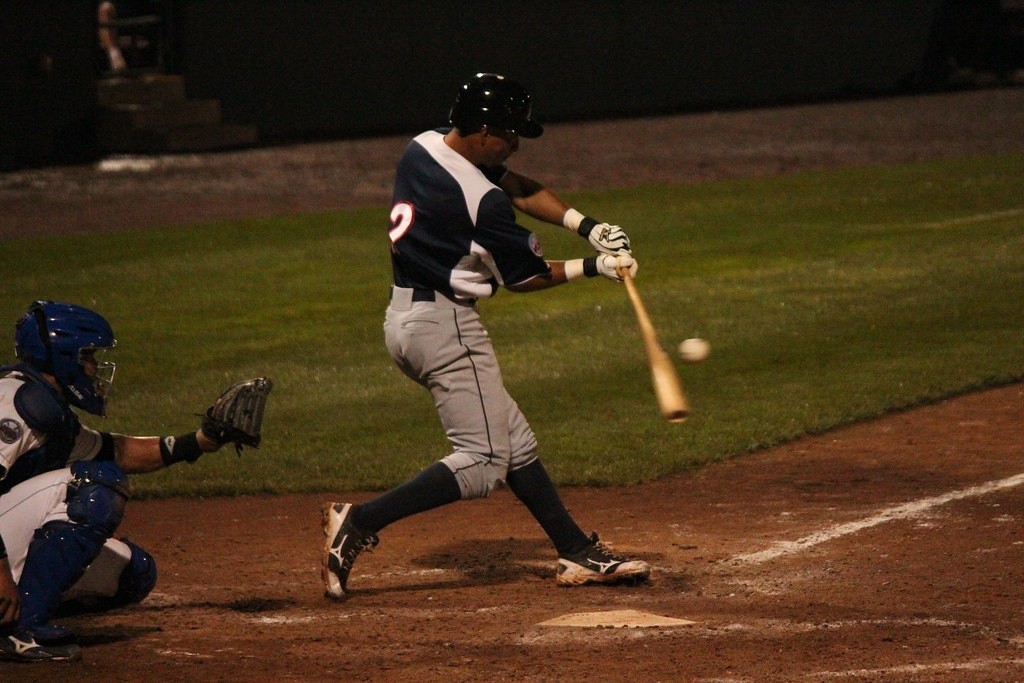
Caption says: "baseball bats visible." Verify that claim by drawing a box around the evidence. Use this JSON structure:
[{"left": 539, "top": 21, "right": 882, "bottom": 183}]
[{"left": 614, "top": 254, "right": 692, "bottom": 424}]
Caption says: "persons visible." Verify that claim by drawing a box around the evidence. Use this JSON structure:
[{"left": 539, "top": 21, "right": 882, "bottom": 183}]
[
  {"left": 318, "top": 70, "right": 652, "bottom": 604},
  {"left": 0, "top": 299, "right": 272, "bottom": 664}
]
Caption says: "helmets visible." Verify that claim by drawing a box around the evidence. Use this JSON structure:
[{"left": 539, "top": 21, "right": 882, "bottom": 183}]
[
  {"left": 449, "top": 68, "right": 545, "bottom": 138},
  {"left": 13, "top": 299, "right": 116, "bottom": 416}
]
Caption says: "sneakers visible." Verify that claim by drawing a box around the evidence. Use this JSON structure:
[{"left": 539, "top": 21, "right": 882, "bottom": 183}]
[
  {"left": 0, "top": 627, "right": 82, "bottom": 662},
  {"left": 556, "top": 531, "right": 650, "bottom": 587},
  {"left": 34, "top": 624, "right": 76, "bottom": 642},
  {"left": 320, "top": 502, "right": 378, "bottom": 600}
]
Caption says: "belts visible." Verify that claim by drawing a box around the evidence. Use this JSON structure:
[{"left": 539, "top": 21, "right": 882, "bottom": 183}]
[{"left": 388, "top": 286, "right": 476, "bottom": 306}]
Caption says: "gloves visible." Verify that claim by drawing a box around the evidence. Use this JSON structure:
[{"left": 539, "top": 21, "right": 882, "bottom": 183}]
[
  {"left": 596, "top": 250, "right": 639, "bottom": 280},
  {"left": 588, "top": 223, "right": 632, "bottom": 255}
]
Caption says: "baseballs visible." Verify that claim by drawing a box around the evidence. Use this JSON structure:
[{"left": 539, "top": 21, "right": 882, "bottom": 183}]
[{"left": 678, "top": 336, "right": 711, "bottom": 366}]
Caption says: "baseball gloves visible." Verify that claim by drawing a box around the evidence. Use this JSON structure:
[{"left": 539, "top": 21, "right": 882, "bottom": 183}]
[{"left": 192, "top": 375, "right": 274, "bottom": 459}]
